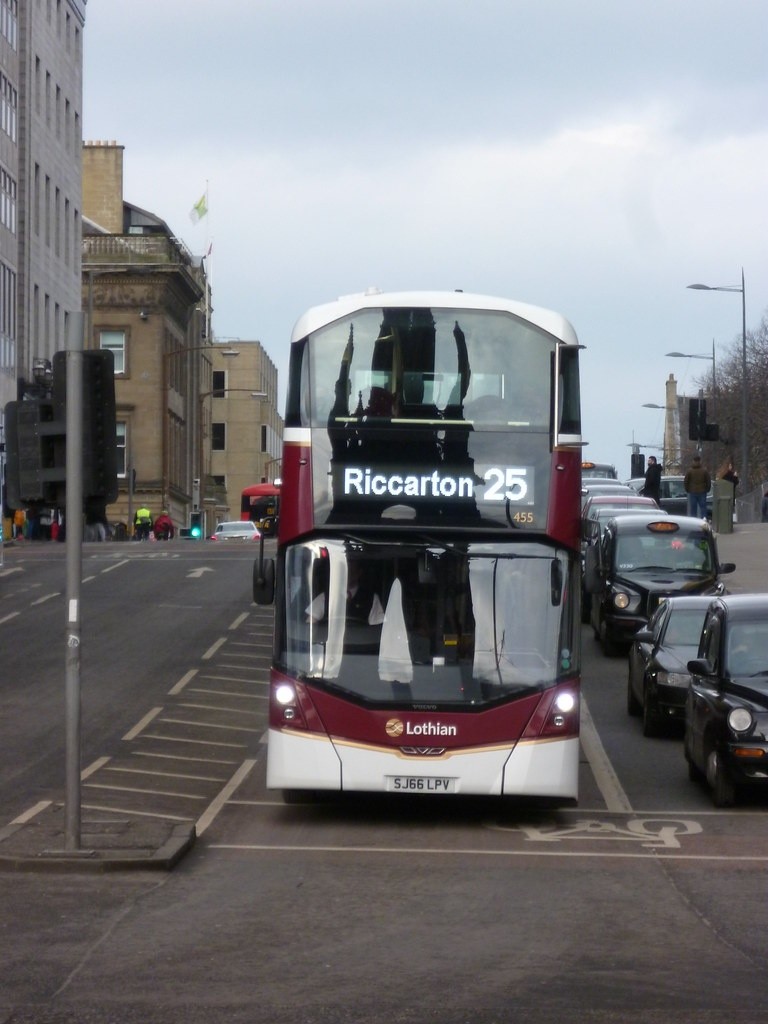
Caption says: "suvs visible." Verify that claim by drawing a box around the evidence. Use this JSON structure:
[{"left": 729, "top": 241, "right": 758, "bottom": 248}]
[
  {"left": 685, "top": 594, "right": 768, "bottom": 809},
  {"left": 584, "top": 514, "right": 737, "bottom": 656}
]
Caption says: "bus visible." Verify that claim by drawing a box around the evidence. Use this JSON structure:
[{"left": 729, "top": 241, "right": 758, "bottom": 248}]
[
  {"left": 253, "top": 292, "right": 602, "bottom": 810},
  {"left": 241, "top": 484, "right": 281, "bottom": 532}
]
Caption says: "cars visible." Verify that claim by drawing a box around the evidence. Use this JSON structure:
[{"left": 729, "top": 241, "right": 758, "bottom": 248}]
[
  {"left": 211, "top": 521, "right": 261, "bottom": 540},
  {"left": 623, "top": 476, "right": 714, "bottom": 520},
  {"left": 627, "top": 596, "right": 718, "bottom": 738},
  {"left": 581, "top": 464, "right": 680, "bottom": 574}
]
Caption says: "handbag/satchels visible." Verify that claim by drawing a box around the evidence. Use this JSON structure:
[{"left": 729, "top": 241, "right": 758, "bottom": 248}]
[{"left": 140, "top": 517, "right": 150, "bottom": 524}]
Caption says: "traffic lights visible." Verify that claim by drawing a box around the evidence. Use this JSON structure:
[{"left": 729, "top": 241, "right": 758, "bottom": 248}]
[{"left": 190, "top": 512, "right": 202, "bottom": 537}]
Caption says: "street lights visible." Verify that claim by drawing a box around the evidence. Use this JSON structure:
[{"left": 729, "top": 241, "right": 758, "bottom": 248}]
[
  {"left": 687, "top": 269, "right": 748, "bottom": 494},
  {"left": 198, "top": 389, "right": 267, "bottom": 507},
  {"left": 161, "top": 345, "right": 239, "bottom": 509},
  {"left": 666, "top": 339, "right": 716, "bottom": 420}
]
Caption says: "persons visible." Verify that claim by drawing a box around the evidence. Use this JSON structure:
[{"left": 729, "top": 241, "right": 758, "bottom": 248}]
[
  {"left": 684, "top": 457, "right": 711, "bottom": 518},
  {"left": 762, "top": 491, "right": 768, "bottom": 523},
  {"left": 643, "top": 456, "right": 662, "bottom": 502},
  {"left": 15, "top": 509, "right": 33, "bottom": 537},
  {"left": 732, "top": 637, "right": 754, "bottom": 663},
  {"left": 721, "top": 462, "right": 739, "bottom": 514},
  {"left": 302, "top": 559, "right": 386, "bottom": 624},
  {"left": 134, "top": 504, "right": 153, "bottom": 540},
  {"left": 154, "top": 510, "right": 174, "bottom": 540}
]
